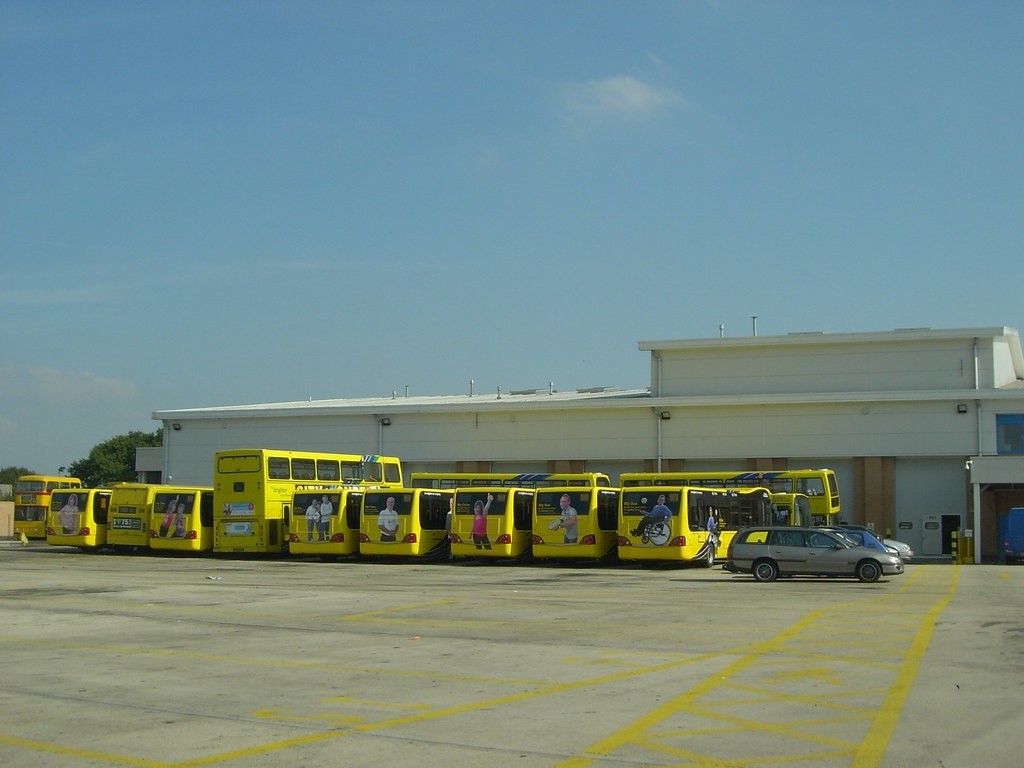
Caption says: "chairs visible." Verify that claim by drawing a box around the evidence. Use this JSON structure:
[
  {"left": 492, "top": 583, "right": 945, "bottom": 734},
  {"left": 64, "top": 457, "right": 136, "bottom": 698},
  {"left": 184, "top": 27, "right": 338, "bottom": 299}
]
[
  {"left": 269, "top": 471, "right": 359, "bottom": 480},
  {"left": 807, "top": 488, "right": 818, "bottom": 496}
]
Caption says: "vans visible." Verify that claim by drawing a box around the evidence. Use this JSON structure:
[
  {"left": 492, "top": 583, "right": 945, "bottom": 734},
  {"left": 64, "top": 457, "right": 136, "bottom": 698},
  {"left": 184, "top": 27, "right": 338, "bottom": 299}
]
[{"left": 1004, "top": 507, "right": 1024, "bottom": 565}]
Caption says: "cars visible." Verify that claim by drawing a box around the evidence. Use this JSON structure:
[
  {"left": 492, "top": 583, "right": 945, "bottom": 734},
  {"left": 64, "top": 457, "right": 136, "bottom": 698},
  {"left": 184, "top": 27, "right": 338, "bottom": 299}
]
[
  {"left": 869, "top": 528, "right": 914, "bottom": 561},
  {"left": 883, "top": 544, "right": 900, "bottom": 557},
  {"left": 834, "top": 526, "right": 889, "bottom": 552},
  {"left": 725, "top": 526, "right": 904, "bottom": 582}
]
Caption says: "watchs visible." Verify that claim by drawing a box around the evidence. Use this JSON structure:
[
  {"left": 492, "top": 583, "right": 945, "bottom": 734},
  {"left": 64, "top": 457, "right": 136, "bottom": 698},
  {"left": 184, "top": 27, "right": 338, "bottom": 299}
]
[{"left": 559, "top": 524, "right": 561, "bottom": 529}]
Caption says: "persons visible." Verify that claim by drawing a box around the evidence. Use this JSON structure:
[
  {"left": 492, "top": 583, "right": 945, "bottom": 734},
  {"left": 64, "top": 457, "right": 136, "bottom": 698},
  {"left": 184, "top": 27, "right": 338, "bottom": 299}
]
[
  {"left": 630, "top": 494, "right": 673, "bottom": 537},
  {"left": 707, "top": 506, "right": 721, "bottom": 549},
  {"left": 56, "top": 494, "right": 78, "bottom": 534},
  {"left": 306, "top": 494, "right": 333, "bottom": 542},
  {"left": 557, "top": 494, "right": 578, "bottom": 543},
  {"left": 377, "top": 497, "right": 399, "bottom": 542},
  {"left": 159, "top": 495, "right": 185, "bottom": 537},
  {"left": 469, "top": 492, "right": 494, "bottom": 549},
  {"left": 445, "top": 498, "right": 453, "bottom": 529}
]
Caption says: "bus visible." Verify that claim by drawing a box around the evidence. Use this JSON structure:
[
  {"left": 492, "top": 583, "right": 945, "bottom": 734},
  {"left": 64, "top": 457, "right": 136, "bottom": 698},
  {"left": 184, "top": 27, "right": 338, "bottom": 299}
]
[
  {"left": 409, "top": 472, "right": 612, "bottom": 488},
  {"left": 289, "top": 490, "right": 360, "bottom": 561},
  {"left": 45, "top": 488, "right": 112, "bottom": 552},
  {"left": 530, "top": 486, "right": 621, "bottom": 561},
  {"left": 211, "top": 448, "right": 405, "bottom": 552},
  {"left": 620, "top": 467, "right": 841, "bottom": 527},
  {"left": 619, "top": 487, "right": 778, "bottom": 569},
  {"left": 450, "top": 486, "right": 532, "bottom": 561},
  {"left": 106, "top": 482, "right": 170, "bottom": 552},
  {"left": 360, "top": 487, "right": 453, "bottom": 561},
  {"left": 13, "top": 475, "right": 82, "bottom": 542},
  {"left": 149, "top": 486, "right": 213, "bottom": 556},
  {"left": 771, "top": 492, "right": 811, "bottom": 528}
]
